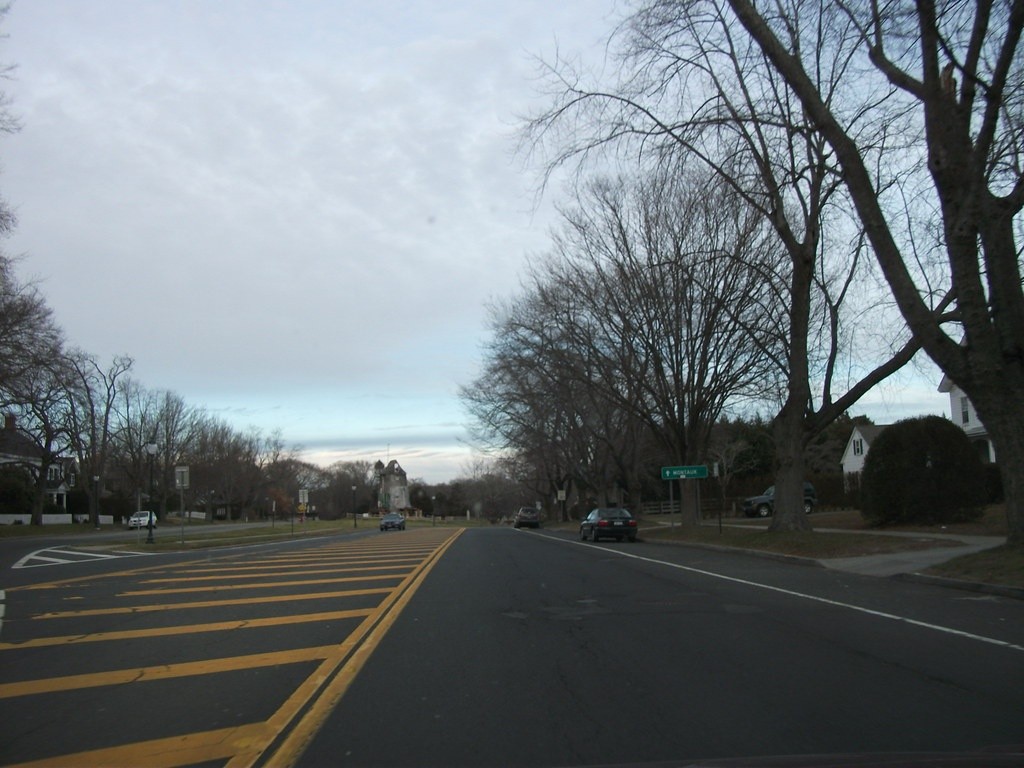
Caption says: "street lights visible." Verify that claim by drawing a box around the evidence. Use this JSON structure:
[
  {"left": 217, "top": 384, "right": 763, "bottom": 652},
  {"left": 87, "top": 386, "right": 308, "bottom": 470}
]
[
  {"left": 146, "top": 439, "right": 160, "bottom": 544},
  {"left": 432, "top": 496, "right": 436, "bottom": 527},
  {"left": 352, "top": 484, "right": 357, "bottom": 529}
]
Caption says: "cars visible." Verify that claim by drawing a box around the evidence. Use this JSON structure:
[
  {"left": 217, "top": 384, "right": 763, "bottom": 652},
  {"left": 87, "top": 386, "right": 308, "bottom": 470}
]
[
  {"left": 742, "top": 482, "right": 817, "bottom": 517},
  {"left": 514, "top": 507, "right": 542, "bottom": 529},
  {"left": 128, "top": 511, "right": 158, "bottom": 530},
  {"left": 579, "top": 508, "right": 638, "bottom": 543}
]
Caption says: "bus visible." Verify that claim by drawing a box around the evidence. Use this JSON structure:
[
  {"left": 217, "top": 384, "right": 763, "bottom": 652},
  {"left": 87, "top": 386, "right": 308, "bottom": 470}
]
[{"left": 379, "top": 513, "right": 406, "bottom": 532}]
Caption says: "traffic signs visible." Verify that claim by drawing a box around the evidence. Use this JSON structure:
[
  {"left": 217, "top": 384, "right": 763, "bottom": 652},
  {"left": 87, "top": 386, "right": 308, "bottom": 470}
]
[{"left": 661, "top": 465, "right": 709, "bottom": 480}]
